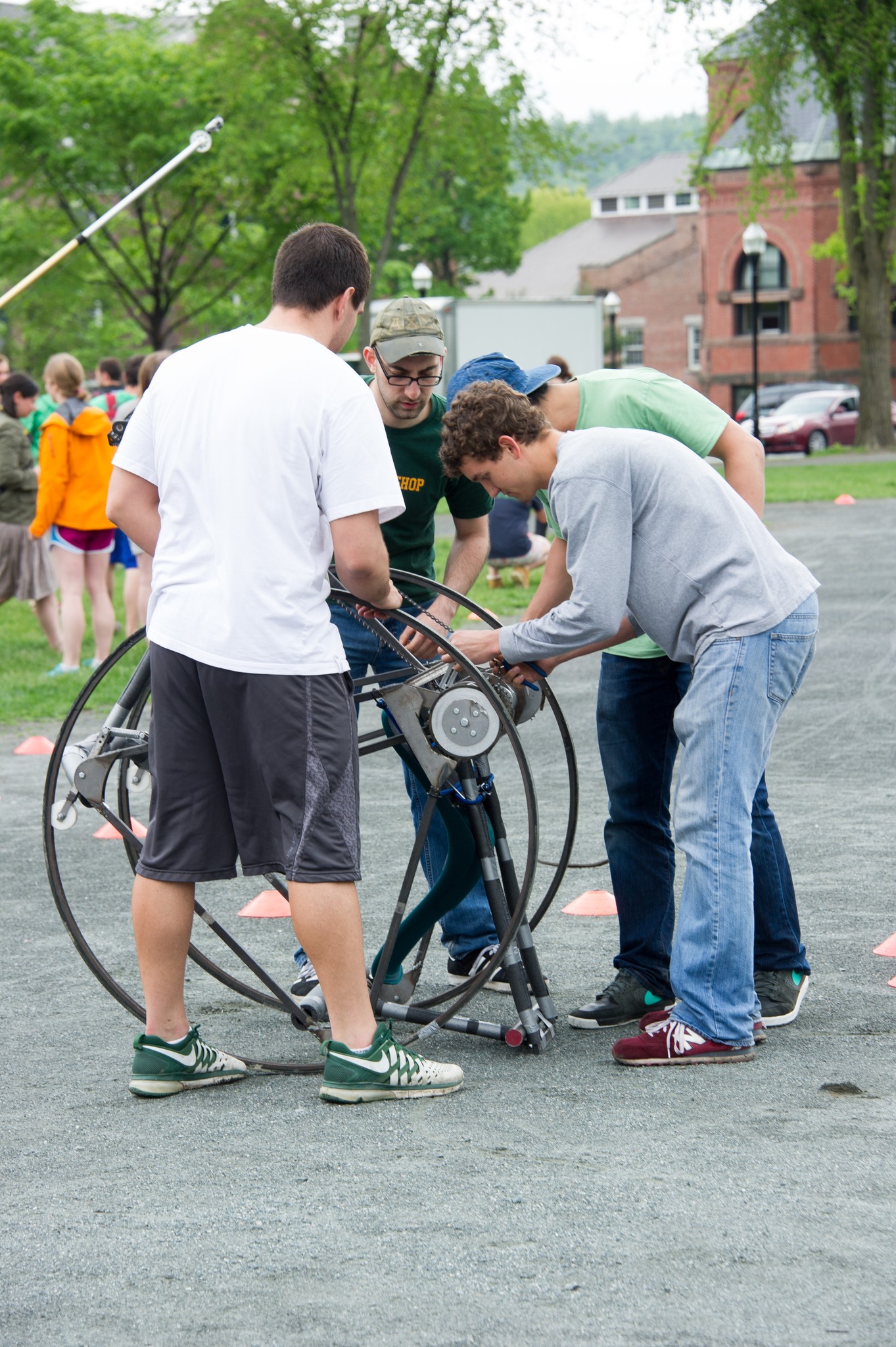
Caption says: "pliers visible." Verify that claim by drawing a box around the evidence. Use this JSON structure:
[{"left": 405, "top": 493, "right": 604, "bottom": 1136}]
[{"left": 501, "top": 659, "right": 547, "bottom": 691}]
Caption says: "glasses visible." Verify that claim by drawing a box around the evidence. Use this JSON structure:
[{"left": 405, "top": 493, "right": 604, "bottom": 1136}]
[{"left": 373, "top": 346, "right": 444, "bottom": 386}]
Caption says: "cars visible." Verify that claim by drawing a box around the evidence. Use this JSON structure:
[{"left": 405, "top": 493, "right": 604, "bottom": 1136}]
[
  {"left": 734, "top": 381, "right": 859, "bottom": 428},
  {"left": 740, "top": 389, "right": 895, "bottom": 454}
]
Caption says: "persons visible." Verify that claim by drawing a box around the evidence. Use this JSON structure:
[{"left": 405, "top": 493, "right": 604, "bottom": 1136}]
[
  {"left": 0, "top": 350, "right": 175, "bottom": 677},
  {"left": 290, "top": 295, "right": 550, "bottom": 995},
  {"left": 444, "top": 351, "right": 810, "bottom": 1029},
  {"left": 545, "top": 356, "right": 573, "bottom": 384},
  {"left": 105, "top": 221, "right": 465, "bottom": 1103},
  {"left": 487, "top": 491, "right": 552, "bottom": 589},
  {"left": 435, "top": 379, "right": 822, "bottom": 1067}
]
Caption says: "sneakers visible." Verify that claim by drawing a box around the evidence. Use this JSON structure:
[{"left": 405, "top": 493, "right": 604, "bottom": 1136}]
[
  {"left": 639, "top": 1005, "right": 766, "bottom": 1041},
  {"left": 128, "top": 1024, "right": 248, "bottom": 1096},
  {"left": 568, "top": 967, "right": 675, "bottom": 1029},
  {"left": 290, "top": 956, "right": 320, "bottom": 1000},
  {"left": 447, "top": 942, "right": 549, "bottom": 994},
  {"left": 755, "top": 965, "right": 809, "bottom": 1026},
  {"left": 319, "top": 1017, "right": 464, "bottom": 1103},
  {"left": 612, "top": 1019, "right": 756, "bottom": 1066}
]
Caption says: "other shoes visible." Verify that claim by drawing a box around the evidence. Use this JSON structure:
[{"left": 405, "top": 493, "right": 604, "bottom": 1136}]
[
  {"left": 488, "top": 576, "right": 503, "bottom": 587},
  {"left": 511, "top": 568, "right": 530, "bottom": 587},
  {"left": 49, "top": 662, "right": 79, "bottom": 674},
  {"left": 80, "top": 659, "right": 102, "bottom": 668}
]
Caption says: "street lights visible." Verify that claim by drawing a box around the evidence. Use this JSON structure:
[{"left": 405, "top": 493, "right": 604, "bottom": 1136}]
[
  {"left": 741, "top": 222, "right": 768, "bottom": 447},
  {"left": 604, "top": 290, "right": 624, "bottom": 370}
]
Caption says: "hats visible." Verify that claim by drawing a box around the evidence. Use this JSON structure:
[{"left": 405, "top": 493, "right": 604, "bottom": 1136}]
[
  {"left": 369, "top": 295, "right": 444, "bottom": 363},
  {"left": 447, "top": 351, "right": 562, "bottom": 412}
]
